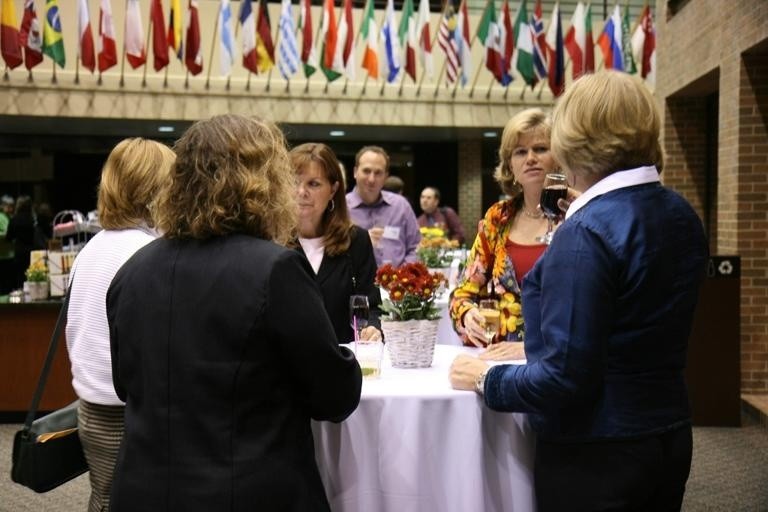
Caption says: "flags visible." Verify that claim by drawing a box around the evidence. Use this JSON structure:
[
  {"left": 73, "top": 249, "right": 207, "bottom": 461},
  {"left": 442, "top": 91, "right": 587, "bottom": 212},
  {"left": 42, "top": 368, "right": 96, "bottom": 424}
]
[
  {"left": 476, "top": 2, "right": 655, "bottom": 98},
  {"left": 216, "top": 2, "right": 471, "bottom": 90},
  {"left": 1, "top": 1, "right": 65, "bottom": 71},
  {"left": 76, "top": 1, "right": 203, "bottom": 76}
]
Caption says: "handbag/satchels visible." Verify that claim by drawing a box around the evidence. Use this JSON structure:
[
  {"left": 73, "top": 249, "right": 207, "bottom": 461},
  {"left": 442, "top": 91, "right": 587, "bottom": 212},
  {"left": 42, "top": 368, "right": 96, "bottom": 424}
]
[{"left": 10, "top": 398, "right": 89, "bottom": 495}]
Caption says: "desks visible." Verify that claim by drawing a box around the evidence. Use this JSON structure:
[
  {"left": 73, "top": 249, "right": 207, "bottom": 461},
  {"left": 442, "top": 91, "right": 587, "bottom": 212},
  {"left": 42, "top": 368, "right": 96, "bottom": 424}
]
[
  {"left": 375, "top": 242, "right": 473, "bottom": 343},
  {"left": 0, "top": 292, "right": 80, "bottom": 413},
  {"left": 309, "top": 343, "right": 541, "bottom": 511}
]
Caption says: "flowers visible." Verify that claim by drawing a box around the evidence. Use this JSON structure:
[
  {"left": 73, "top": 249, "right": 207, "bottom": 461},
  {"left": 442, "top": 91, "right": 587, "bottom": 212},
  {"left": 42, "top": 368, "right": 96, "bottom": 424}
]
[
  {"left": 413, "top": 225, "right": 461, "bottom": 265},
  {"left": 369, "top": 260, "right": 447, "bottom": 319},
  {"left": 23, "top": 252, "right": 50, "bottom": 282}
]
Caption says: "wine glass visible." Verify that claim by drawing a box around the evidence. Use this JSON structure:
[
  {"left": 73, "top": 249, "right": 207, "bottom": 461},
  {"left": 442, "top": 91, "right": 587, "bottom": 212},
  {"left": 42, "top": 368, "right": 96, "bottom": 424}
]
[
  {"left": 533, "top": 173, "right": 568, "bottom": 244},
  {"left": 348, "top": 294, "right": 369, "bottom": 340},
  {"left": 480, "top": 301, "right": 500, "bottom": 352}
]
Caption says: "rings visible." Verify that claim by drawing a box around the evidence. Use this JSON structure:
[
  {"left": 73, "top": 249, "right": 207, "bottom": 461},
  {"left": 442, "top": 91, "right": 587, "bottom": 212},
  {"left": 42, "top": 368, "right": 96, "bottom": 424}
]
[{"left": 465, "top": 329, "right": 472, "bottom": 334}]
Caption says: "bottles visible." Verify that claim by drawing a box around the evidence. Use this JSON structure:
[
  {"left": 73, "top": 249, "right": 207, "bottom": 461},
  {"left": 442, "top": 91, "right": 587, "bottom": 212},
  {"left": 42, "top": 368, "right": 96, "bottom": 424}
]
[{"left": 458, "top": 244, "right": 468, "bottom": 276}]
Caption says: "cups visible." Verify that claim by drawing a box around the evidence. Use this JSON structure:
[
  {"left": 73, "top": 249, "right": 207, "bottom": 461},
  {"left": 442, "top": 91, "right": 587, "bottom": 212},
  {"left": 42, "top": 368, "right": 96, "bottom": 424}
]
[{"left": 350, "top": 340, "right": 383, "bottom": 378}]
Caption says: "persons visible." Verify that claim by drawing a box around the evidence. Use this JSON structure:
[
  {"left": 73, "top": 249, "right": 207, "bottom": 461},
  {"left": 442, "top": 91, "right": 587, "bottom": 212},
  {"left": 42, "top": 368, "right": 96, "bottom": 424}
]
[
  {"left": 415, "top": 187, "right": 465, "bottom": 248},
  {"left": 385, "top": 174, "right": 406, "bottom": 196},
  {"left": 0, "top": 194, "right": 17, "bottom": 296},
  {"left": 105, "top": 112, "right": 360, "bottom": 511},
  {"left": 288, "top": 143, "right": 384, "bottom": 345},
  {"left": 62, "top": 138, "right": 179, "bottom": 511},
  {"left": 451, "top": 69, "right": 708, "bottom": 512},
  {"left": 37, "top": 204, "right": 55, "bottom": 238},
  {"left": 7, "top": 195, "right": 39, "bottom": 288},
  {"left": 450, "top": 107, "right": 572, "bottom": 362},
  {"left": 341, "top": 146, "right": 423, "bottom": 269}
]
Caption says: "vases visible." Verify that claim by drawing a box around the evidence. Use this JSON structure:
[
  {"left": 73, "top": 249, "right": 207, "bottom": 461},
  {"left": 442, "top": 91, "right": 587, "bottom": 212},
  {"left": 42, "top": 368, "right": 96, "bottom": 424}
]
[{"left": 27, "top": 282, "right": 49, "bottom": 300}]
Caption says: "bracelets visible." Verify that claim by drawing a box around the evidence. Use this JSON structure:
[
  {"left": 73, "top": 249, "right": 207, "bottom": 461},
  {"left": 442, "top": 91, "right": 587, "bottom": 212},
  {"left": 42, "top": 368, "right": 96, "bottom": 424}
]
[{"left": 473, "top": 366, "right": 491, "bottom": 395}]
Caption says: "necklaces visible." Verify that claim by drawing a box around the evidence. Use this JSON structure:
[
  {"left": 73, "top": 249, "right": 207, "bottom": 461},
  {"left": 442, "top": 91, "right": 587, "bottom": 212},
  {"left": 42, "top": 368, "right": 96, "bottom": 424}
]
[{"left": 522, "top": 203, "right": 544, "bottom": 218}]
